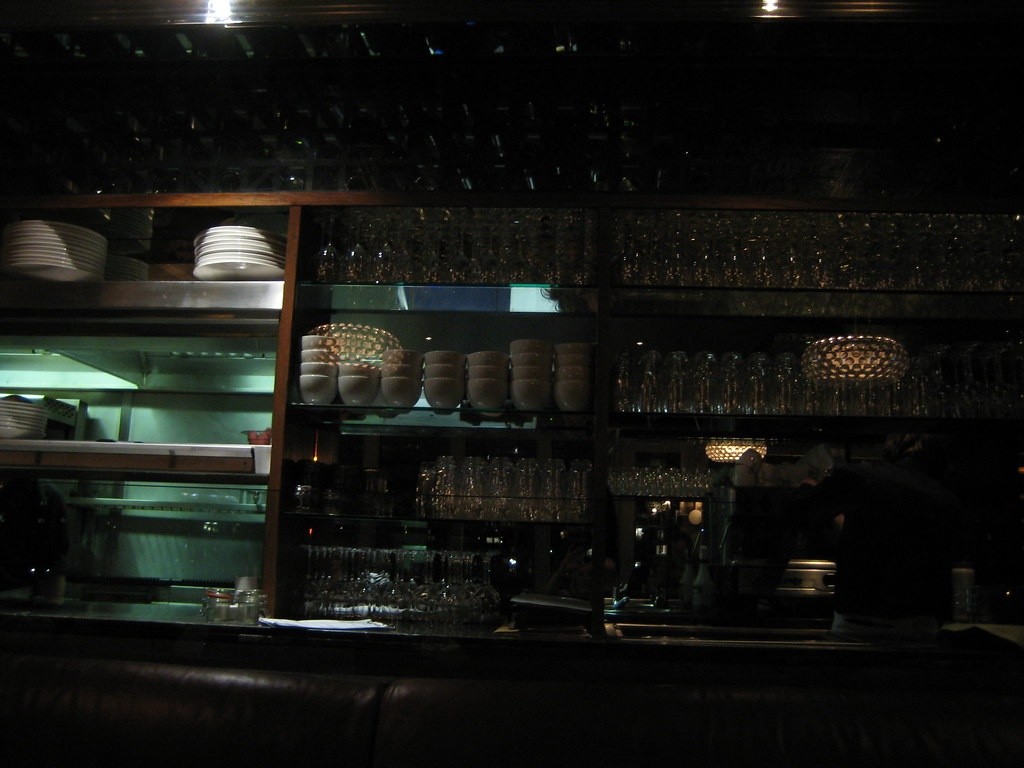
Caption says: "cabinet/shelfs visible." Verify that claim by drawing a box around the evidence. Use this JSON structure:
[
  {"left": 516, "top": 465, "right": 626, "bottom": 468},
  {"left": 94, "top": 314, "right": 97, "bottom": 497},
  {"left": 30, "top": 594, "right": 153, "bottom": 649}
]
[{"left": 0, "top": 192, "right": 1024, "bottom": 670}]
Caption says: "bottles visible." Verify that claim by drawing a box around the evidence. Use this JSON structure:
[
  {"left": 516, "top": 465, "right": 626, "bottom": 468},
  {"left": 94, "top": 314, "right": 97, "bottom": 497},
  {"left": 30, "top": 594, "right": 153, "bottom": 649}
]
[
  {"left": 691, "top": 545, "right": 714, "bottom": 614},
  {"left": 677, "top": 544, "right": 697, "bottom": 611}
]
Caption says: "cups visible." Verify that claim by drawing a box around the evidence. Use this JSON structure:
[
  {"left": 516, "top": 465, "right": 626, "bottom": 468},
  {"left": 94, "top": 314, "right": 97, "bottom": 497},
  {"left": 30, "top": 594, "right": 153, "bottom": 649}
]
[
  {"left": 606, "top": 347, "right": 920, "bottom": 417},
  {"left": 609, "top": 466, "right": 713, "bottom": 498},
  {"left": 233, "top": 575, "right": 257, "bottom": 604},
  {"left": 416, "top": 455, "right": 589, "bottom": 521}
]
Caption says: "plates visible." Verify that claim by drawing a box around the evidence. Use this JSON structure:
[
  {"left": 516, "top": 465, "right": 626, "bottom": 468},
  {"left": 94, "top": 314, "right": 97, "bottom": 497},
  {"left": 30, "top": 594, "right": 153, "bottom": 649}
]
[
  {"left": 110, "top": 254, "right": 149, "bottom": 280},
  {"left": 193, "top": 222, "right": 287, "bottom": 281},
  {"left": 0, "top": 400, "right": 47, "bottom": 438},
  {"left": 98, "top": 209, "right": 153, "bottom": 253},
  {"left": 2, "top": 220, "right": 107, "bottom": 281}
]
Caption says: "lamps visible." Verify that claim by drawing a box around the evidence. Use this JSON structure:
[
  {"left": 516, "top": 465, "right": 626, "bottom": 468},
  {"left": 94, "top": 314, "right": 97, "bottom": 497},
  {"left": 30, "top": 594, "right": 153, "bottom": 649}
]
[{"left": 802, "top": 317, "right": 911, "bottom": 384}]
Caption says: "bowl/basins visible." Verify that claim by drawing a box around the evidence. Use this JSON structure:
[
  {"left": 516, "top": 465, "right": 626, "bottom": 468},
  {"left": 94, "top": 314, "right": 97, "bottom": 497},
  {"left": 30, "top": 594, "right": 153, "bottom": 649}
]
[
  {"left": 382, "top": 349, "right": 422, "bottom": 407},
  {"left": 424, "top": 349, "right": 465, "bottom": 409},
  {"left": 338, "top": 361, "right": 380, "bottom": 405},
  {"left": 550, "top": 342, "right": 592, "bottom": 410},
  {"left": 299, "top": 333, "right": 337, "bottom": 403},
  {"left": 466, "top": 351, "right": 509, "bottom": 414},
  {"left": 509, "top": 338, "right": 553, "bottom": 409}
]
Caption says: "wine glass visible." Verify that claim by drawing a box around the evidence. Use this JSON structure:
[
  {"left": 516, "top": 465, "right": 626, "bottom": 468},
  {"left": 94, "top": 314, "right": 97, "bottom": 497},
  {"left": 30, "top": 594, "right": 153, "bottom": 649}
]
[
  {"left": 366, "top": 467, "right": 395, "bottom": 518},
  {"left": 920, "top": 341, "right": 1024, "bottom": 418},
  {"left": 604, "top": 212, "right": 1022, "bottom": 291},
  {"left": 301, "top": 543, "right": 503, "bottom": 622},
  {"left": 315, "top": 207, "right": 595, "bottom": 287}
]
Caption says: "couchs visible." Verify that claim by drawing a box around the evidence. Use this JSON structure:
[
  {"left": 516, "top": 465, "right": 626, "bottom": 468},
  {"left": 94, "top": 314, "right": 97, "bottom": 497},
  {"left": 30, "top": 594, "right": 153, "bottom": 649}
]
[{"left": 0, "top": 656, "right": 1024, "bottom": 768}]
